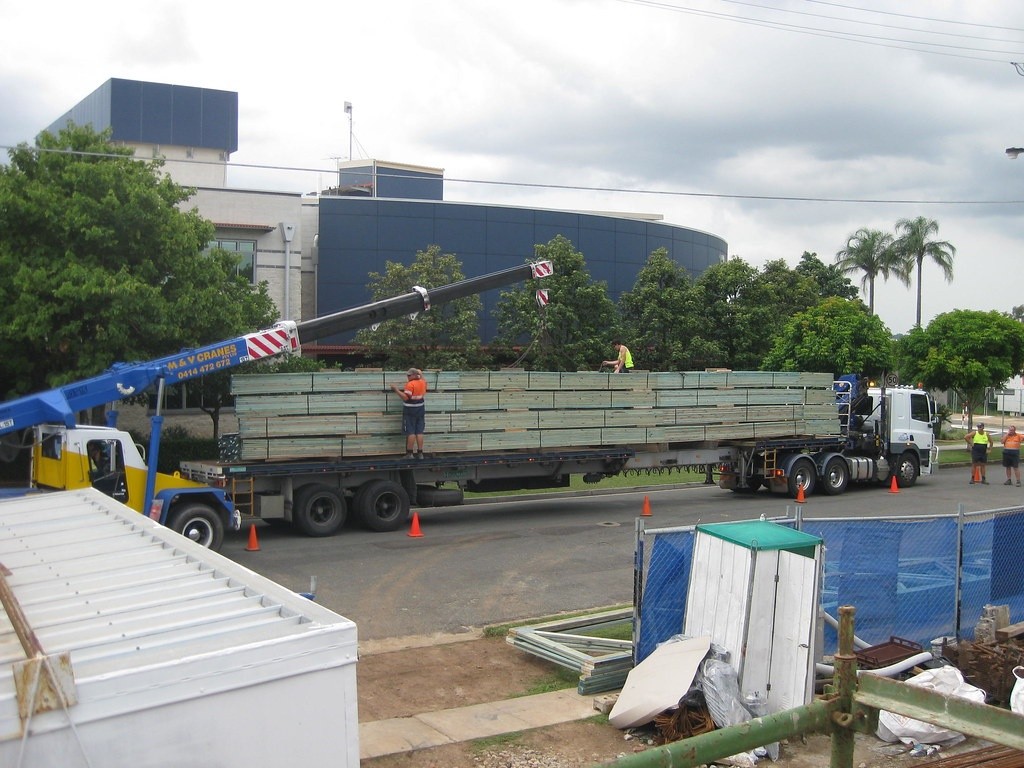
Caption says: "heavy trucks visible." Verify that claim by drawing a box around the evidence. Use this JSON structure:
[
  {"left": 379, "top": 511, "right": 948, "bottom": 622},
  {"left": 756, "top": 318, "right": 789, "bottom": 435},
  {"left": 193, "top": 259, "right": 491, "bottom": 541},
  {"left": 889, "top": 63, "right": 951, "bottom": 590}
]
[{"left": 179, "top": 369, "right": 939, "bottom": 499}]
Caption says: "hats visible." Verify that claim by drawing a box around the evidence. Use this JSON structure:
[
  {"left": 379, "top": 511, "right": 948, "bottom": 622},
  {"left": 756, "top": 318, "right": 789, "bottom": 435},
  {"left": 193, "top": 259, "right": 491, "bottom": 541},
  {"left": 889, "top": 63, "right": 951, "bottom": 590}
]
[
  {"left": 977, "top": 422, "right": 984, "bottom": 427},
  {"left": 407, "top": 368, "right": 418, "bottom": 375}
]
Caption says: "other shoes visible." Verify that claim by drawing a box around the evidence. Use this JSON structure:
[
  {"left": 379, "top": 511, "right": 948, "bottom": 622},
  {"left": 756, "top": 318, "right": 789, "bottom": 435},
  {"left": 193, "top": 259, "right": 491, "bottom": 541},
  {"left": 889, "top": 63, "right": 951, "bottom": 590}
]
[
  {"left": 414, "top": 453, "right": 423, "bottom": 460},
  {"left": 1004, "top": 480, "right": 1012, "bottom": 485},
  {"left": 1016, "top": 481, "right": 1021, "bottom": 486},
  {"left": 402, "top": 454, "right": 413, "bottom": 459},
  {"left": 970, "top": 478, "right": 976, "bottom": 484},
  {"left": 982, "top": 478, "right": 989, "bottom": 484}
]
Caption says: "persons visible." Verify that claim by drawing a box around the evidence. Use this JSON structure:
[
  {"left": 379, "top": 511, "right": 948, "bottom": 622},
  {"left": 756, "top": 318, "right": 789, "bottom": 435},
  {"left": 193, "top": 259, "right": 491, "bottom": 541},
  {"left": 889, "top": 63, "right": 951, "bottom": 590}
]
[
  {"left": 391, "top": 368, "right": 427, "bottom": 459},
  {"left": 1001, "top": 425, "right": 1024, "bottom": 486},
  {"left": 965, "top": 422, "right": 993, "bottom": 484},
  {"left": 90, "top": 440, "right": 122, "bottom": 499},
  {"left": 603, "top": 340, "right": 633, "bottom": 373}
]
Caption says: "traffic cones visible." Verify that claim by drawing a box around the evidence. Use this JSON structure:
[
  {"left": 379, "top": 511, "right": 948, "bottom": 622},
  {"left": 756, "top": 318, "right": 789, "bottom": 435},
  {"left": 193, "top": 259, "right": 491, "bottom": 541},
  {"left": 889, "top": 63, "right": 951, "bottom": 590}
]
[
  {"left": 406, "top": 513, "right": 425, "bottom": 538},
  {"left": 973, "top": 466, "right": 981, "bottom": 482},
  {"left": 887, "top": 476, "right": 900, "bottom": 493},
  {"left": 244, "top": 524, "right": 260, "bottom": 551},
  {"left": 793, "top": 484, "right": 807, "bottom": 502},
  {"left": 640, "top": 496, "right": 654, "bottom": 516}
]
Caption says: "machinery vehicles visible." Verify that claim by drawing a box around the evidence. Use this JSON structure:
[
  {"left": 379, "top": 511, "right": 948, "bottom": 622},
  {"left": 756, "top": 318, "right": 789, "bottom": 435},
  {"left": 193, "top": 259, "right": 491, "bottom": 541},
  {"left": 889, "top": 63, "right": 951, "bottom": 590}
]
[{"left": 0, "top": 256, "right": 553, "bottom": 554}]
